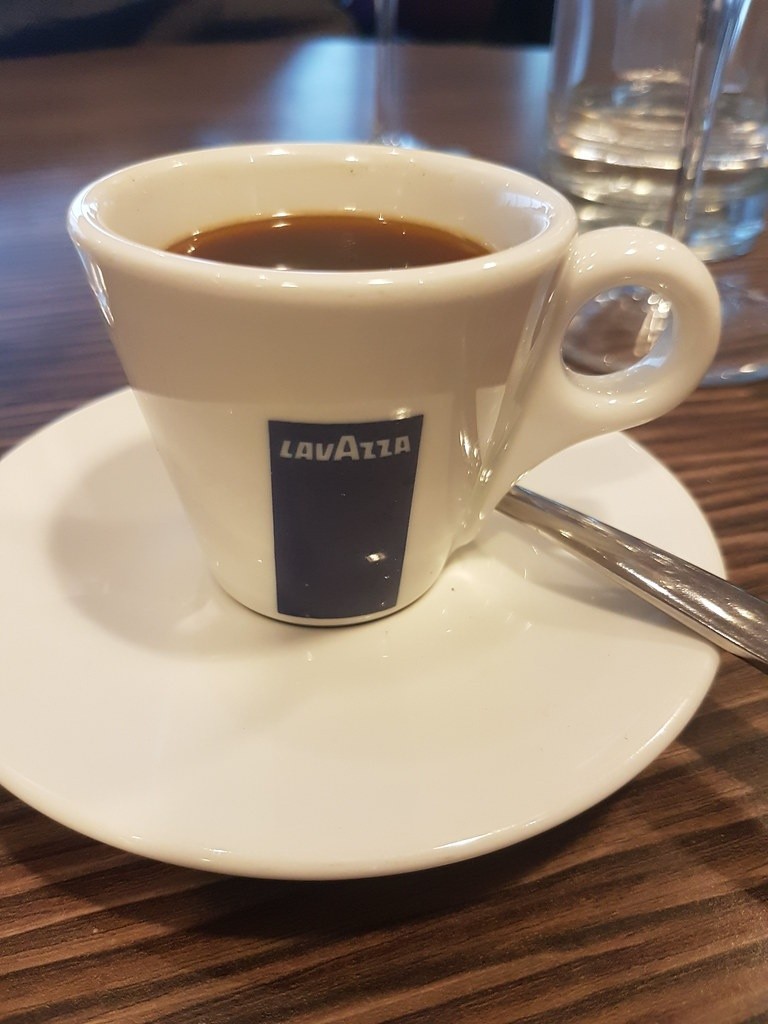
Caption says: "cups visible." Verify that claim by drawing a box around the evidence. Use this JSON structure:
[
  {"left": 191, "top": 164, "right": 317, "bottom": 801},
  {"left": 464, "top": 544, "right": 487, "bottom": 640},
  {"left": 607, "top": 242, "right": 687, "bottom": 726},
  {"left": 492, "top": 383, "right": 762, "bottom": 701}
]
[
  {"left": 547, "top": 0, "right": 767, "bottom": 263},
  {"left": 66, "top": 142, "right": 721, "bottom": 626}
]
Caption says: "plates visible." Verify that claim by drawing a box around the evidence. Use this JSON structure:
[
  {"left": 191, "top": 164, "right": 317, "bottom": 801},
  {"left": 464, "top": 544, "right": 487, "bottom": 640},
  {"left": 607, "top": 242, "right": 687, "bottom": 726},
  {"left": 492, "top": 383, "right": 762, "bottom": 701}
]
[{"left": 0, "top": 388, "right": 728, "bottom": 881}]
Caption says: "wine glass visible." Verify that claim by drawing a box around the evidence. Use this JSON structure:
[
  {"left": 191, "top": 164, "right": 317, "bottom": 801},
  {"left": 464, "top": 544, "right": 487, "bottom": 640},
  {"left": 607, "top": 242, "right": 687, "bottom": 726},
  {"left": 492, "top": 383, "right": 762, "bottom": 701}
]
[{"left": 562, "top": 0, "right": 767, "bottom": 384}]
